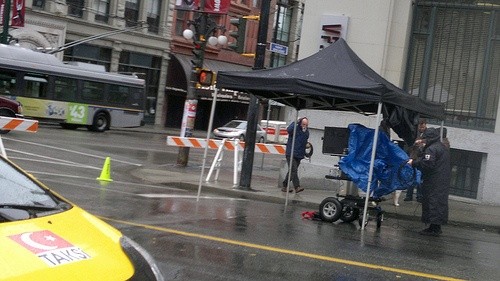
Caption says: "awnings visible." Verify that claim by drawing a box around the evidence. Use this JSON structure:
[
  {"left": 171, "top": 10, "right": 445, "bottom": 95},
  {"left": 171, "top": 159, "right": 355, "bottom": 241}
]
[{"left": 172, "top": 52, "right": 288, "bottom": 108}]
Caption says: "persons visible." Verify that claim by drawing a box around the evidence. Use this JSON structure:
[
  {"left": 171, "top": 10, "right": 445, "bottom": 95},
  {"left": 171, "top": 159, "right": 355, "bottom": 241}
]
[
  {"left": 403, "top": 118, "right": 428, "bottom": 203},
  {"left": 435, "top": 127, "right": 452, "bottom": 152},
  {"left": 281, "top": 116, "right": 310, "bottom": 193},
  {"left": 406, "top": 126, "right": 452, "bottom": 236}
]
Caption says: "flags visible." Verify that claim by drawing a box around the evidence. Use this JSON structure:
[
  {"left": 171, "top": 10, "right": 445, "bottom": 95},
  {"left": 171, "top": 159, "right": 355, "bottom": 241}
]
[
  {"left": 0, "top": 0, "right": 5, "bottom": 25},
  {"left": 203, "top": 0, "right": 230, "bottom": 15},
  {"left": 9, "top": 0, "right": 26, "bottom": 29},
  {"left": 174, "top": 0, "right": 199, "bottom": 12}
]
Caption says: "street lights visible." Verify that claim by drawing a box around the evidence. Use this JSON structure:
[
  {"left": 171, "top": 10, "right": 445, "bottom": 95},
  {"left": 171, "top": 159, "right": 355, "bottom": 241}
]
[{"left": 176, "top": 23, "right": 227, "bottom": 166}]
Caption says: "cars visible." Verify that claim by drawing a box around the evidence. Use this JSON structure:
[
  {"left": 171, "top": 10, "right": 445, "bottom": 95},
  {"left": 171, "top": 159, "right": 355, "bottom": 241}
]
[
  {"left": 213, "top": 120, "right": 267, "bottom": 144},
  {"left": 0, "top": 96, "right": 23, "bottom": 134},
  {"left": 0, "top": 133, "right": 164, "bottom": 281}
]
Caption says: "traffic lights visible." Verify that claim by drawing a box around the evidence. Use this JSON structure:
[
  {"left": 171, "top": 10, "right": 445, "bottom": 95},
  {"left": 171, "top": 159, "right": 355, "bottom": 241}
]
[
  {"left": 199, "top": 71, "right": 212, "bottom": 85},
  {"left": 227, "top": 17, "right": 246, "bottom": 55}
]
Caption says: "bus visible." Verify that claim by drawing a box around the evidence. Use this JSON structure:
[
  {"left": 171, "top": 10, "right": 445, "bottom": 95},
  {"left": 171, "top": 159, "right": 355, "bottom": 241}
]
[{"left": 0, "top": 42, "right": 146, "bottom": 133}]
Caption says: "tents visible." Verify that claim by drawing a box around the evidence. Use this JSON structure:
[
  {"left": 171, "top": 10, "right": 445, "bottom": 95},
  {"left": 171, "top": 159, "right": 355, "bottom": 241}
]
[{"left": 194, "top": 37, "right": 446, "bottom": 232}]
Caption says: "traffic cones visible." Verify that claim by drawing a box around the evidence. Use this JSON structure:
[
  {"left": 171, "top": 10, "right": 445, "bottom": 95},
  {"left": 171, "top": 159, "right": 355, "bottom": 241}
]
[{"left": 96, "top": 156, "right": 113, "bottom": 181}]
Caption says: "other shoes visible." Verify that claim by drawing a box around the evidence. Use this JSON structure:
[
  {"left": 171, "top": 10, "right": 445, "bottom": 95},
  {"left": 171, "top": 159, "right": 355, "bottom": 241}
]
[
  {"left": 295, "top": 187, "right": 304, "bottom": 193},
  {"left": 404, "top": 196, "right": 413, "bottom": 201},
  {"left": 282, "top": 187, "right": 293, "bottom": 192},
  {"left": 417, "top": 197, "right": 423, "bottom": 203},
  {"left": 421, "top": 227, "right": 443, "bottom": 236}
]
